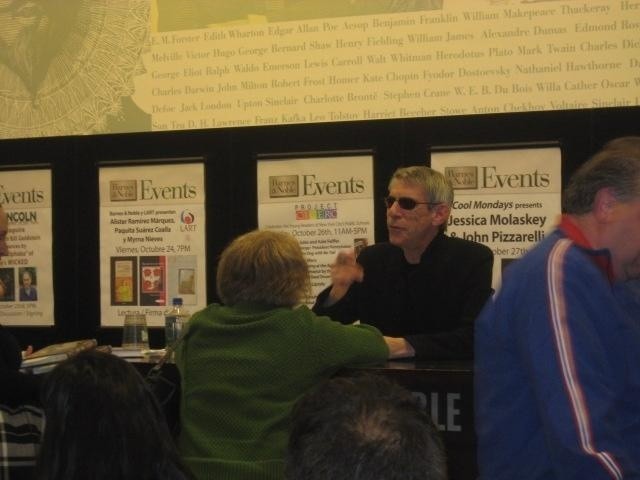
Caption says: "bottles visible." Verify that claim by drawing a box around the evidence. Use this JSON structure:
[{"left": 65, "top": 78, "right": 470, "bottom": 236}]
[{"left": 164, "top": 297, "right": 192, "bottom": 363}]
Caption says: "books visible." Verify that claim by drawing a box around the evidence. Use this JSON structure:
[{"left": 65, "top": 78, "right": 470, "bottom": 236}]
[{"left": 18, "top": 339, "right": 173, "bottom": 376}]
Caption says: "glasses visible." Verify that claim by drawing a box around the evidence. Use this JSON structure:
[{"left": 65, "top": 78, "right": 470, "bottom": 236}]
[{"left": 384, "top": 196, "right": 442, "bottom": 212}]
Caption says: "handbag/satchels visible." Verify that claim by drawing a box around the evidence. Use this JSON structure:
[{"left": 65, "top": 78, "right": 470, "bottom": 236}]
[{"left": 144, "top": 360, "right": 180, "bottom": 436}]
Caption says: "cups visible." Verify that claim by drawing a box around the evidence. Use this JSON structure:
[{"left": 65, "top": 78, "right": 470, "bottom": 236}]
[{"left": 122, "top": 315, "right": 150, "bottom": 352}]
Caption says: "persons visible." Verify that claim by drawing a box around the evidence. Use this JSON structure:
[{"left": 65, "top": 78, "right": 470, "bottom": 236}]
[
  {"left": 42, "top": 347, "right": 195, "bottom": 479},
  {"left": 18, "top": 271, "right": 37, "bottom": 301},
  {"left": 287, "top": 371, "right": 447, "bottom": 478},
  {"left": 0, "top": 204, "right": 32, "bottom": 357},
  {"left": 310, "top": 164, "right": 493, "bottom": 365},
  {"left": 173, "top": 228, "right": 387, "bottom": 476},
  {"left": 473, "top": 136, "right": 640, "bottom": 479},
  {"left": 353, "top": 239, "right": 366, "bottom": 256}
]
